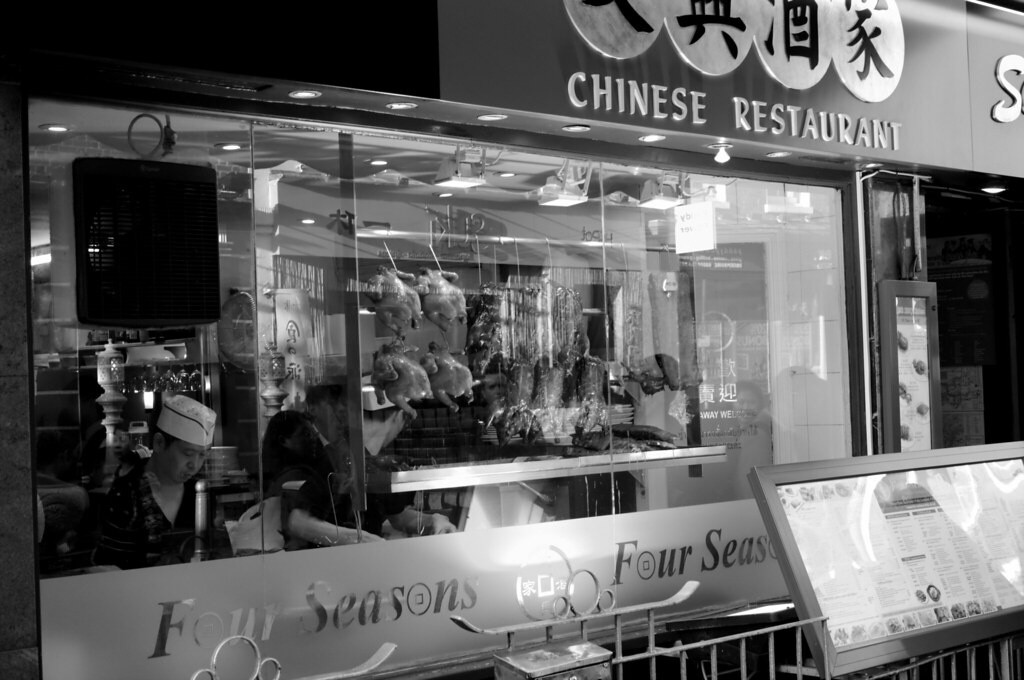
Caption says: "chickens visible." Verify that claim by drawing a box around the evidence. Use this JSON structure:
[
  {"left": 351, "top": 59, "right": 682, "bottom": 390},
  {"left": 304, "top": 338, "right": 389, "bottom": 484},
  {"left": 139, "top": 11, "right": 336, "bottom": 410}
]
[
  {"left": 371, "top": 342, "right": 434, "bottom": 419},
  {"left": 410, "top": 267, "right": 468, "bottom": 331},
  {"left": 364, "top": 266, "right": 423, "bottom": 341},
  {"left": 420, "top": 342, "right": 474, "bottom": 413}
]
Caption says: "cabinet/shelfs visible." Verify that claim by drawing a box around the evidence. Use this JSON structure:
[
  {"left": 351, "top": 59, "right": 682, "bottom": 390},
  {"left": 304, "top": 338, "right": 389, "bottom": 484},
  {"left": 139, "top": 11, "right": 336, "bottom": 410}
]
[{"left": 35, "top": 316, "right": 223, "bottom": 483}]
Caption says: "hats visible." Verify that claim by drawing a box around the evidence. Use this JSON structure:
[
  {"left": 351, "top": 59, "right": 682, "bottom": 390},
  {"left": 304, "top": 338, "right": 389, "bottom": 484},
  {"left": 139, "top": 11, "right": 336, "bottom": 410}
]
[
  {"left": 156, "top": 394, "right": 218, "bottom": 447},
  {"left": 362, "top": 385, "right": 411, "bottom": 411}
]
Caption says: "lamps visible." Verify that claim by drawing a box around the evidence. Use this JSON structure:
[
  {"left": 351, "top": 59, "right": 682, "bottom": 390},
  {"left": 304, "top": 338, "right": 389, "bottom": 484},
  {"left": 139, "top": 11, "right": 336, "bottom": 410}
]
[
  {"left": 638, "top": 177, "right": 682, "bottom": 209},
  {"left": 536, "top": 176, "right": 589, "bottom": 207},
  {"left": 433, "top": 157, "right": 484, "bottom": 190}
]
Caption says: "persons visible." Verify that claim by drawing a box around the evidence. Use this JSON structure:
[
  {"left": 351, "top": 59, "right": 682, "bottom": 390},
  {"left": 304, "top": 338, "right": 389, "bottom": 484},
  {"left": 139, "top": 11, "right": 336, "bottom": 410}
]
[
  {"left": 89, "top": 395, "right": 234, "bottom": 571},
  {"left": 259, "top": 383, "right": 457, "bottom": 552},
  {"left": 456, "top": 368, "right": 572, "bottom": 532}
]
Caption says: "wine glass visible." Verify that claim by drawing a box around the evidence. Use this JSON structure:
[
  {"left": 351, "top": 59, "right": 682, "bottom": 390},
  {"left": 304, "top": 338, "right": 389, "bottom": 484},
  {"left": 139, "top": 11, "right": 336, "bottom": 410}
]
[{"left": 119, "top": 361, "right": 206, "bottom": 393}]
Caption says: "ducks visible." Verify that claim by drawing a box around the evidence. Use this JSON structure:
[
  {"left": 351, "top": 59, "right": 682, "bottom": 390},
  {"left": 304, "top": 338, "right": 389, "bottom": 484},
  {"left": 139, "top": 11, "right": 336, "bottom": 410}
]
[
  {"left": 525, "top": 354, "right": 567, "bottom": 446},
  {"left": 484, "top": 361, "right": 537, "bottom": 450},
  {"left": 565, "top": 352, "right": 610, "bottom": 455}
]
